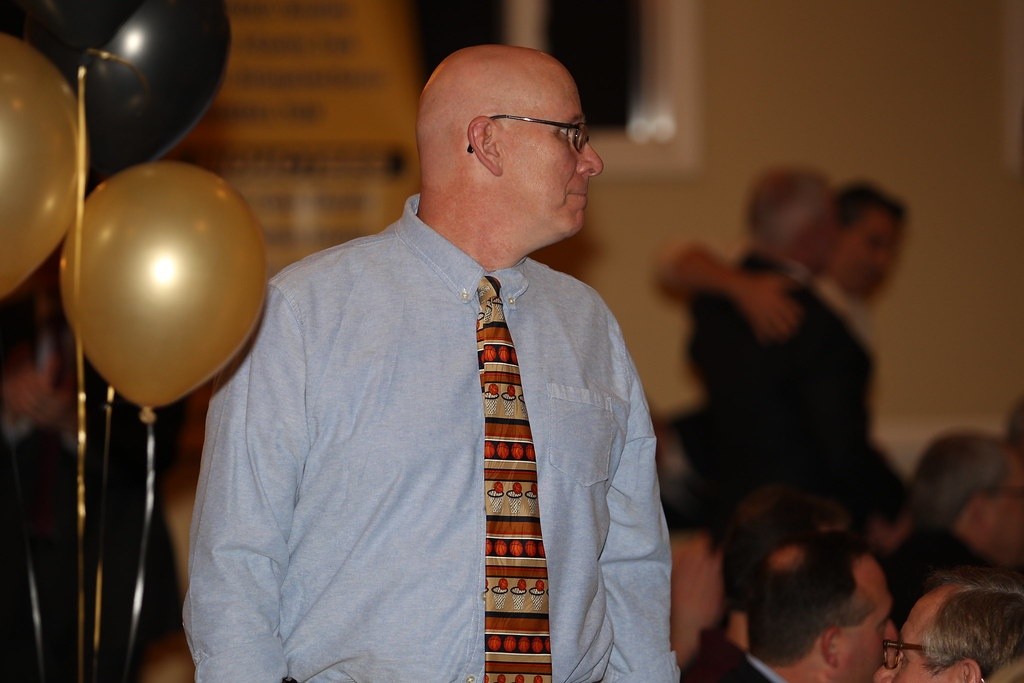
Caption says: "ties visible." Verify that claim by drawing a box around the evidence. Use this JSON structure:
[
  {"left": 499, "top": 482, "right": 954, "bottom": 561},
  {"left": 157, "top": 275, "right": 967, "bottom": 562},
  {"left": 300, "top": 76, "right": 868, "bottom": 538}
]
[{"left": 478, "top": 271, "right": 552, "bottom": 683}]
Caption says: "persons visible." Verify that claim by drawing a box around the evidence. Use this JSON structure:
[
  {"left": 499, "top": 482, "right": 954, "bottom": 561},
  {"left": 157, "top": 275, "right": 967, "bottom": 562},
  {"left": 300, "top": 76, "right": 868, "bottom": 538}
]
[
  {"left": 183, "top": 44, "right": 681, "bottom": 683},
  {"left": 653, "top": 179, "right": 910, "bottom": 343},
  {"left": 684, "top": 165, "right": 877, "bottom": 599},
  {"left": 652, "top": 421, "right": 900, "bottom": 683},
  {"left": 0, "top": 289, "right": 197, "bottom": 683},
  {"left": 877, "top": 398, "right": 1024, "bottom": 683}
]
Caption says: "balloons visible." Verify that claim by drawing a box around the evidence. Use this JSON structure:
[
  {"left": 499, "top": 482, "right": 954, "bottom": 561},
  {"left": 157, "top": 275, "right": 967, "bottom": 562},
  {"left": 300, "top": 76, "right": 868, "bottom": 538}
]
[
  {"left": 0, "top": 33, "right": 90, "bottom": 304},
  {"left": 0, "top": 0, "right": 231, "bottom": 174},
  {"left": 61, "top": 160, "right": 266, "bottom": 424}
]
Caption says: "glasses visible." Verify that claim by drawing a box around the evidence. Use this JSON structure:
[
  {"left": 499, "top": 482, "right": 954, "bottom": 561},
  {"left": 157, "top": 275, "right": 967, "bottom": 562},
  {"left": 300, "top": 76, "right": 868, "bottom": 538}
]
[
  {"left": 883, "top": 639, "right": 982, "bottom": 683},
  {"left": 467, "top": 112, "right": 589, "bottom": 157}
]
